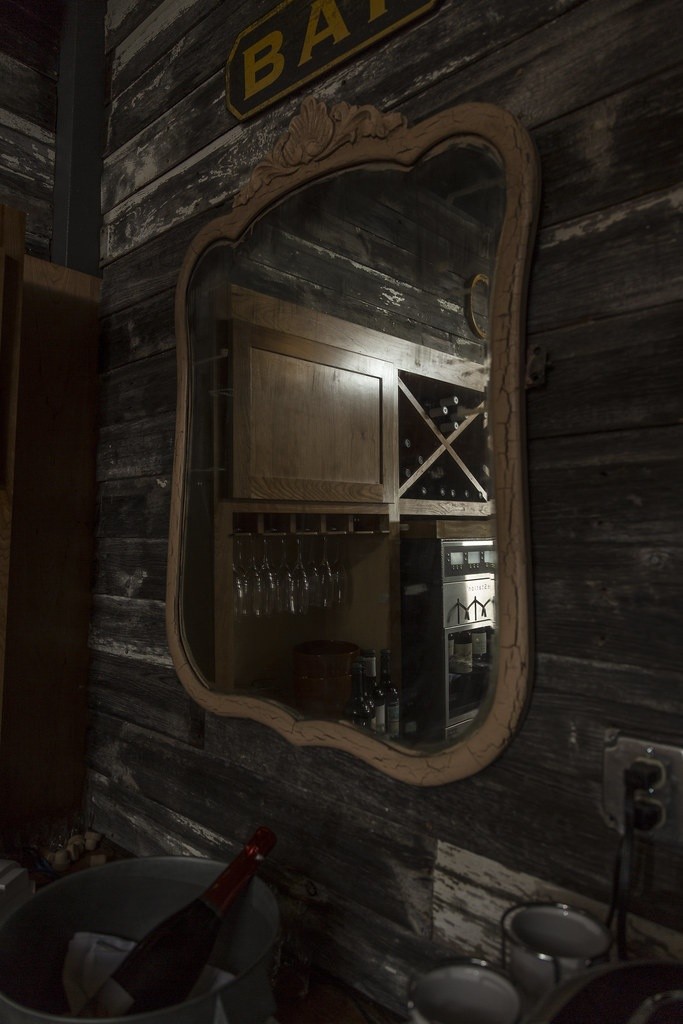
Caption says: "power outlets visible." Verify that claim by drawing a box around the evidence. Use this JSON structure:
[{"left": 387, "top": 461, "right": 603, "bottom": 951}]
[{"left": 596, "top": 736, "right": 683, "bottom": 848}]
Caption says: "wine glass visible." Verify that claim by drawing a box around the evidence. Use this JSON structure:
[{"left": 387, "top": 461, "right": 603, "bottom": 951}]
[{"left": 233, "top": 529, "right": 348, "bottom": 616}]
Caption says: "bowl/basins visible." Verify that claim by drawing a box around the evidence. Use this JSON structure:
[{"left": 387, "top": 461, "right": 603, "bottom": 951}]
[
  {"left": 295, "top": 675, "right": 351, "bottom": 719},
  {"left": 291, "top": 640, "right": 360, "bottom": 677}
]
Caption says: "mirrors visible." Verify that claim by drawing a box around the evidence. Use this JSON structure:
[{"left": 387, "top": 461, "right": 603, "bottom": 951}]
[{"left": 166, "top": 99, "right": 539, "bottom": 791}]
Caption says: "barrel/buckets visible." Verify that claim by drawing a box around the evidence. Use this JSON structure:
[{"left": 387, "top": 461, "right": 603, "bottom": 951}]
[{"left": 0, "top": 857, "right": 281, "bottom": 1024}]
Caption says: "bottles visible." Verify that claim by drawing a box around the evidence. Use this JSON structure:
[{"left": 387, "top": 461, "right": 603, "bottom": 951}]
[
  {"left": 76, "top": 826, "right": 276, "bottom": 1015},
  {"left": 399, "top": 396, "right": 488, "bottom": 500},
  {"left": 378, "top": 650, "right": 401, "bottom": 742},
  {"left": 343, "top": 661, "right": 376, "bottom": 732},
  {"left": 405, "top": 957, "right": 524, "bottom": 1023},
  {"left": 360, "top": 649, "right": 387, "bottom": 737},
  {"left": 448, "top": 627, "right": 491, "bottom": 694},
  {"left": 502, "top": 903, "right": 613, "bottom": 1009}
]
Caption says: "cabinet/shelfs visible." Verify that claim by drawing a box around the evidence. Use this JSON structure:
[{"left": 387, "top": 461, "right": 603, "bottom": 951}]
[{"left": 201, "top": 282, "right": 491, "bottom": 738}]
